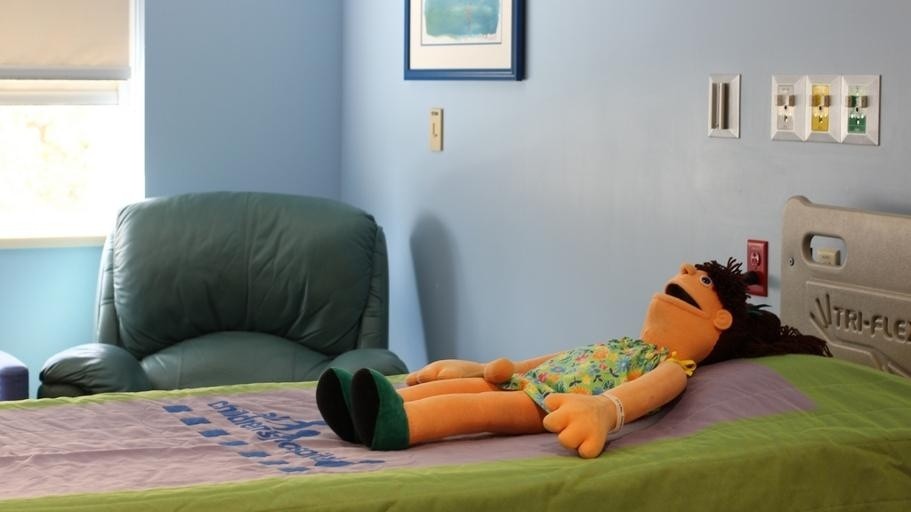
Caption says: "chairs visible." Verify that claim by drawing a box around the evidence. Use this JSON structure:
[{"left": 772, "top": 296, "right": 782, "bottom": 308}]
[{"left": 38, "top": 189, "right": 408, "bottom": 398}]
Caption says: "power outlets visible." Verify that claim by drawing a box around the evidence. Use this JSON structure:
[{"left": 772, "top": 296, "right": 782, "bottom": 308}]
[{"left": 771, "top": 73, "right": 881, "bottom": 144}]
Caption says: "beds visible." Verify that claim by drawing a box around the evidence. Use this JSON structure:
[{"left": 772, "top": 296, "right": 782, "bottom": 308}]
[{"left": 3, "top": 196, "right": 911, "bottom": 506}]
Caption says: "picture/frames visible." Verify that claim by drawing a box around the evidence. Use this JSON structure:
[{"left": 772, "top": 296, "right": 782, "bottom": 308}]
[{"left": 403, "top": 0, "right": 524, "bottom": 82}]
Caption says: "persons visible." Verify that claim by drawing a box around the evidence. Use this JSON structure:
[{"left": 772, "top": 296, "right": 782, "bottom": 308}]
[{"left": 312, "top": 256, "right": 836, "bottom": 461}]
